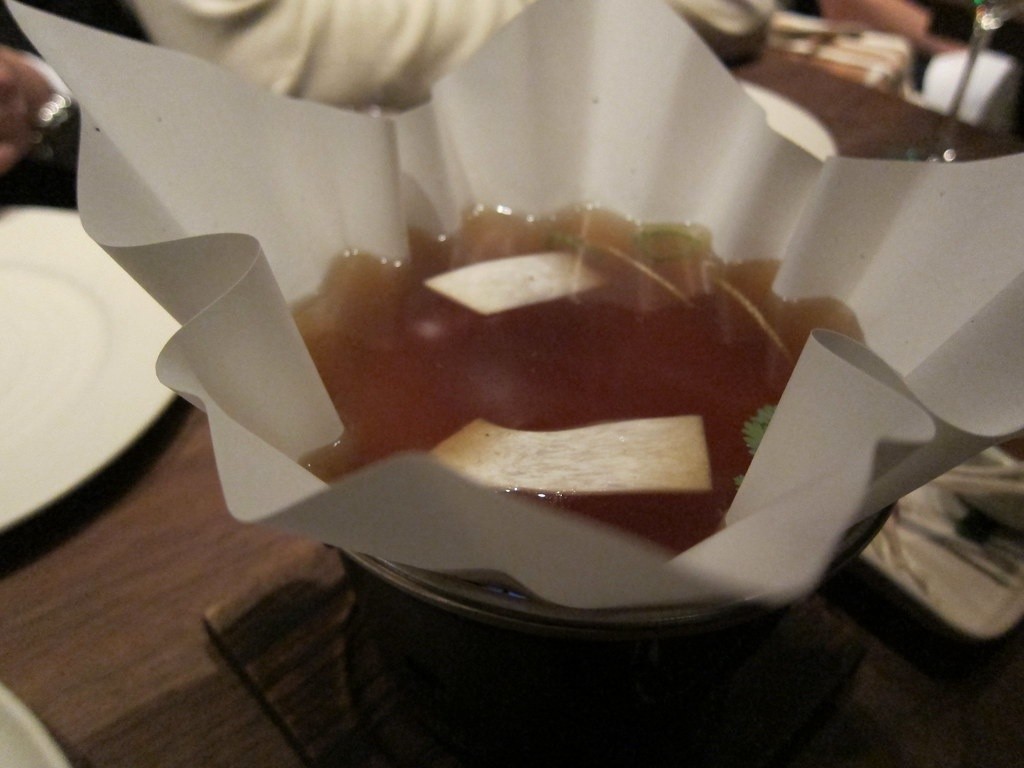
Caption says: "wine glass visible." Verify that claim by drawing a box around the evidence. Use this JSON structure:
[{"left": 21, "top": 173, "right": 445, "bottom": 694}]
[{"left": 905, "top": 0, "right": 1023, "bottom": 162}]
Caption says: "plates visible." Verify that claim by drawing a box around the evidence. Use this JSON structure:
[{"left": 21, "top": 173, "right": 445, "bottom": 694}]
[
  {"left": 0, "top": 204, "right": 182, "bottom": 530},
  {"left": 0, "top": 681, "right": 75, "bottom": 768},
  {"left": 741, "top": 79, "right": 837, "bottom": 161},
  {"left": 859, "top": 446, "right": 1024, "bottom": 640}
]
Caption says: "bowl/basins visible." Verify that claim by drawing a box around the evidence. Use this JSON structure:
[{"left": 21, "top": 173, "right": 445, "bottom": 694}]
[{"left": 343, "top": 500, "right": 898, "bottom": 638}]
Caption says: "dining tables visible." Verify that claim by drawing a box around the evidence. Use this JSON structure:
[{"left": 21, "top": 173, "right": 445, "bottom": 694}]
[{"left": 0, "top": 48, "right": 1023, "bottom": 767}]
